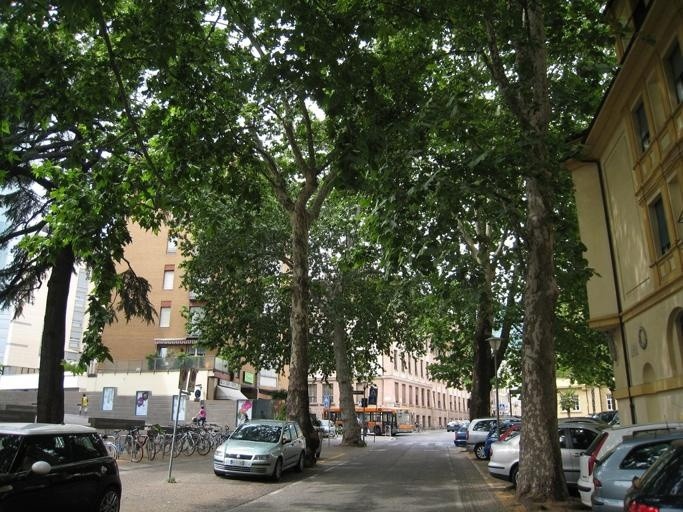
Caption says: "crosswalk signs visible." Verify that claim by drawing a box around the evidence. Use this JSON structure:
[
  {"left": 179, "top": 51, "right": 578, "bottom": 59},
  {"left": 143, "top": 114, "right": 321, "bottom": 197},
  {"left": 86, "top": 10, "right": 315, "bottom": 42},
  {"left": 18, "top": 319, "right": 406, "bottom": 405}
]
[{"left": 370, "top": 388, "right": 377, "bottom": 395}]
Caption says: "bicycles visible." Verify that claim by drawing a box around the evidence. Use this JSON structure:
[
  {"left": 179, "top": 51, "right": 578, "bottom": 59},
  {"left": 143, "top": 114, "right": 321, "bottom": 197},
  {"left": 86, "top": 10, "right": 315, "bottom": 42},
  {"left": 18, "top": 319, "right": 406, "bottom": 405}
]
[{"left": 99, "top": 424, "right": 230, "bottom": 463}]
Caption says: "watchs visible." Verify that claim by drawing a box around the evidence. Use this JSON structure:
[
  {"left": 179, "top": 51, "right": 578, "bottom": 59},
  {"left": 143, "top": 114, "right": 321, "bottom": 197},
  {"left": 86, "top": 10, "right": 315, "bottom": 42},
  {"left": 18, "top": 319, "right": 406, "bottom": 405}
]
[{"left": 79, "top": 394, "right": 88, "bottom": 416}]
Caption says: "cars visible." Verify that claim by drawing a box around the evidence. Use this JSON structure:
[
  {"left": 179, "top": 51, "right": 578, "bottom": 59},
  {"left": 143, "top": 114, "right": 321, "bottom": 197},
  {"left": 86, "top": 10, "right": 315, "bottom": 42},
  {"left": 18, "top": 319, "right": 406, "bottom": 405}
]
[
  {"left": 446, "top": 411, "right": 682, "bottom": 512},
  {"left": 313, "top": 420, "right": 336, "bottom": 437},
  {"left": 214, "top": 419, "right": 306, "bottom": 482},
  {"left": 309, "top": 416, "right": 322, "bottom": 461},
  {"left": 0, "top": 423, "right": 121, "bottom": 512}
]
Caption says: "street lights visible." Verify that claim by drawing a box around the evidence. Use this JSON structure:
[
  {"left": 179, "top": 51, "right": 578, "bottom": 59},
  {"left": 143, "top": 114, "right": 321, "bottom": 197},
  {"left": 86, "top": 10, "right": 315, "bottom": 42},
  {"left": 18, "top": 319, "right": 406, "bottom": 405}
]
[
  {"left": 361, "top": 381, "right": 368, "bottom": 447},
  {"left": 486, "top": 335, "right": 503, "bottom": 440}
]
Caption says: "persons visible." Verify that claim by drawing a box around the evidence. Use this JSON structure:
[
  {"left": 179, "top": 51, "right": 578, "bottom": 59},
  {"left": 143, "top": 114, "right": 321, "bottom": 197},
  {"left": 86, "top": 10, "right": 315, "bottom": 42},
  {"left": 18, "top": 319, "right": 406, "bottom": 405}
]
[{"left": 196, "top": 405, "right": 206, "bottom": 426}]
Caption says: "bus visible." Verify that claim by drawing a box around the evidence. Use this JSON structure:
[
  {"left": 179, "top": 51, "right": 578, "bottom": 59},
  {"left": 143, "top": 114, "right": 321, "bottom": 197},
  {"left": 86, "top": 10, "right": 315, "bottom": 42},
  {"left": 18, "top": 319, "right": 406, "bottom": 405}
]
[{"left": 322, "top": 407, "right": 416, "bottom": 436}]
